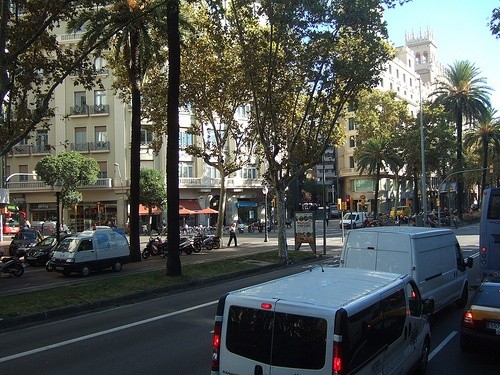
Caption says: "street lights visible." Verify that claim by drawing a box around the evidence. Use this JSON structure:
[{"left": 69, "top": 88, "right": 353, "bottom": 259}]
[
  {"left": 54, "top": 180, "right": 63, "bottom": 243},
  {"left": 262, "top": 185, "right": 269, "bottom": 242},
  {"left": 436, "top": 168, "right": 490, "bottom": 227},
  {"left": 112, "top": 162, "right": 126, "bottom": 234}
]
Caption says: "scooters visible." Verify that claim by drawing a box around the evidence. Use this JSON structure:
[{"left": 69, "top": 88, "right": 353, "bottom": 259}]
[
  {"left": 142, "top": 232, "right": 221, "bottom": 259},
  {"left": 0, "top": 247, "right": 27, "bottom": 277}
]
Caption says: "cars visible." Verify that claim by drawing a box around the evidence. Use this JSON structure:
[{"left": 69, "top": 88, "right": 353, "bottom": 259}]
[
  {"left": 9, "top": 230, "right": 44, "bottom": 257},
  {"left": 31, "top": 221, "right": 56, "bottom": 232},
  {"left": 27, "top": 236, "right": 57, "bottom": 267},
  {"left": 460, "top": 282, "right": 500, "bottom": 350}
]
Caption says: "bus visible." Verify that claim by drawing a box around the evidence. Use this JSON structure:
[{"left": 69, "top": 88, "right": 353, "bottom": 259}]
[
  {"left": 479, "top": 188, "right": 500, "bottom": 283},
  {"left": 2, "top": 210, "right": 26, "bottom": 233}
]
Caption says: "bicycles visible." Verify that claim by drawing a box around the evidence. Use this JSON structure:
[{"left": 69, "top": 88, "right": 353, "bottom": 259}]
[
  {"left": 412, "top": 208, "right": 459, "bottom": 229},
  {"left": 163, "top": 225, "right": 217, "bottom": 235}
]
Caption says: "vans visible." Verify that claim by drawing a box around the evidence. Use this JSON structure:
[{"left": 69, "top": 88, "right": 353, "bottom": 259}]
[
  {"left": 51, "top": 229, "right": 131, "bottom": 278},
  {"left": 339, "top": 225, "right": 474, "bottom": 319},
  {"left": 390, "top": 206, "right": 410, "bottom": 218},
  {"left": 211, "top": 265, "right": 435, "bottom": 375}
]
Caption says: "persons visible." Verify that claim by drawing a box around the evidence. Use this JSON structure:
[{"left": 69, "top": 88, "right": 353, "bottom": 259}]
[{"left": 227, "top": 223, "right": 239, "bottom": 247}]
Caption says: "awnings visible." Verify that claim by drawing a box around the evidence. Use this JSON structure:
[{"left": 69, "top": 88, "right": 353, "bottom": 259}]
[{"left": 137, "top": 199, "right": 219, "bottom": 216}]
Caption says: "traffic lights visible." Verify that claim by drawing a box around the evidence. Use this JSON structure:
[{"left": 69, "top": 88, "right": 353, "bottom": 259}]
[{"left": 345, "top": 197, "right": 350, "bottom": 210}]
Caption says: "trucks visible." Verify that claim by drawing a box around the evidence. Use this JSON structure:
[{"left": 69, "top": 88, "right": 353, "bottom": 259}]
[{"left": 338, "top": 212, "right": 373, "bottom": 229}]
